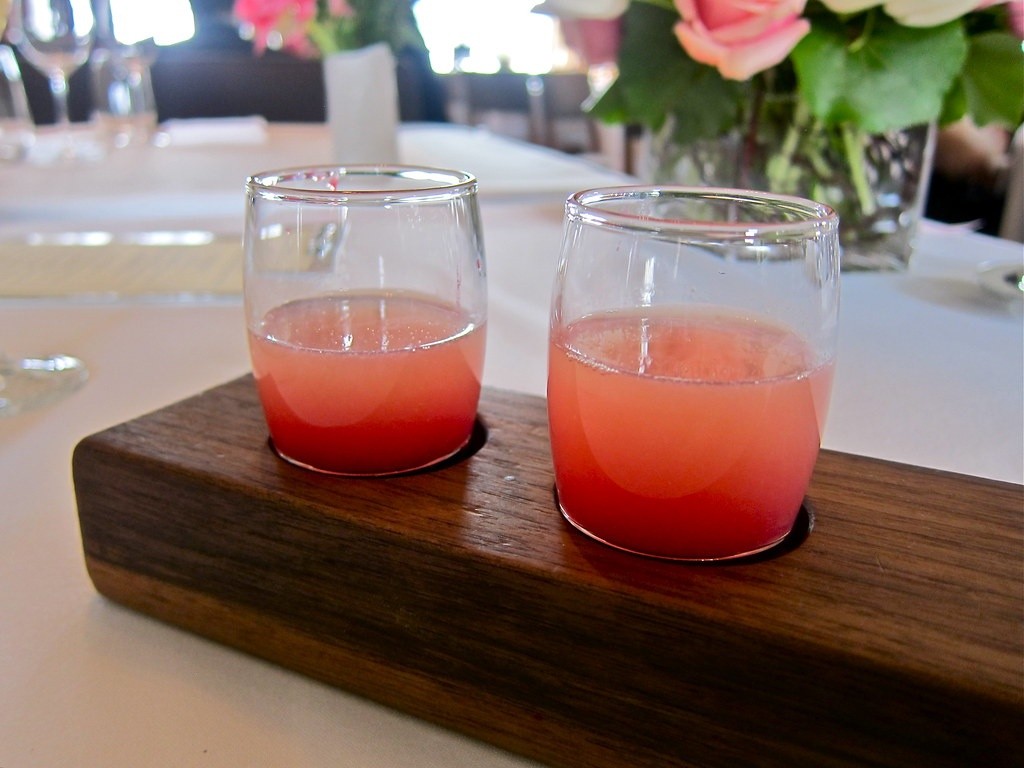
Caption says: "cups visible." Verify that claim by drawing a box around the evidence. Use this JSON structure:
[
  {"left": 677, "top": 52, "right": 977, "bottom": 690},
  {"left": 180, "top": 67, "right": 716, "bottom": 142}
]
[
  {"left": 549, "top": 185, "right": 842, "bottom": 562},
  {"left": 242, "top": 164, "right": 488, "bottom": 476}
]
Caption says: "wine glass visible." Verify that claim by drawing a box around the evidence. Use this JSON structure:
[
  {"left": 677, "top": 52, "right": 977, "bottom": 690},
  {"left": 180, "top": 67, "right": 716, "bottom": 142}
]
[{"left": 1, "top": 0, "right": 170, "bottom": 168}]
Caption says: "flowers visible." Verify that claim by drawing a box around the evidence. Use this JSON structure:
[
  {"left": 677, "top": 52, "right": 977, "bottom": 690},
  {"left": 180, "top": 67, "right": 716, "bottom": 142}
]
[
  {"left": 530, "top": 0, "right": 1022, "bottom": 235},
  {"left": 233, "top": 2, "right": 416, "bottom": 60}
]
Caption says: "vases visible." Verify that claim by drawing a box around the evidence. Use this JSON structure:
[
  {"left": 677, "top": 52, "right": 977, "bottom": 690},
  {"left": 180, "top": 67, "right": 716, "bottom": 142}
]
[
  {"left": 321, "top": 43, "right": 400, "bottom": 168},
  {"left": 645, "top": 109, "right": 941, "bottom": 272}
]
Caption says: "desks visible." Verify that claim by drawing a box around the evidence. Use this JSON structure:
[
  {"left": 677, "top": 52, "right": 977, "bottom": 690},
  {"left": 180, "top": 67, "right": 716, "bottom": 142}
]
[{"left": 2, "top": 118, "right": 1023, "bottom": 767}]
